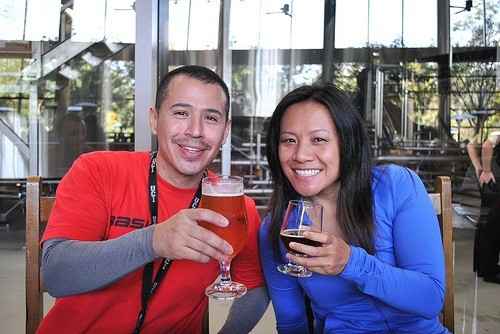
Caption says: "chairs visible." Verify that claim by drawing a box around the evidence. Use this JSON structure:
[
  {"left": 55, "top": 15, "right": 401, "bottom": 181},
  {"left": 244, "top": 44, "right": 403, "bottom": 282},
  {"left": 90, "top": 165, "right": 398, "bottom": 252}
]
[
  {"left": 306, "top": 175, "right": 454, "bottom": 334},
  {"left": 26, "top": 176, "right": 209, "bottom": 333}
]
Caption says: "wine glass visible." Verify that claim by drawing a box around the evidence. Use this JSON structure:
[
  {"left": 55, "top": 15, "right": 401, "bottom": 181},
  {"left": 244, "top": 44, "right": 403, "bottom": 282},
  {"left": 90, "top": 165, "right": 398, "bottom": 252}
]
[
  {"left": 277, "top": 201, "right": 323, "bottom": 277},
  {"left": 201, "top": 175, "right": 247, "bottom": 300}
]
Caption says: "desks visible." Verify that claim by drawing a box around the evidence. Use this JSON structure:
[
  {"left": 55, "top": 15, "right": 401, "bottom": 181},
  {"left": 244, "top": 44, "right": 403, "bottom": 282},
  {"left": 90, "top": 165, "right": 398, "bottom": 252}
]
[{"left": 241, "top": 138, "right": 500, "bottom": 193}]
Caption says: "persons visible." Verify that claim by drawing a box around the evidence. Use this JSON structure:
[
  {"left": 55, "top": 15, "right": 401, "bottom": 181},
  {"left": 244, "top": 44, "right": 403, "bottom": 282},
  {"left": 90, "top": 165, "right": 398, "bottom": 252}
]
[
  {"left": 350, "top": 67, "right": 417, "bottom": 133},
  {"left": 47, "top": 113, "right": 106, "bottom": 190},
  {"left": 467, "top": 130, "right": 500, "bottom": 284},
  {"left": 257, "top": 84, "right": 454, "bottom": 334},
  {"left": 35, "top": 65, "right": 271, "bottom": 334}
]
